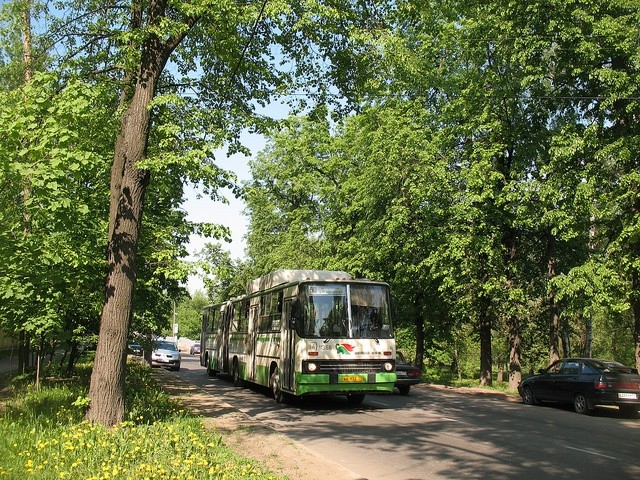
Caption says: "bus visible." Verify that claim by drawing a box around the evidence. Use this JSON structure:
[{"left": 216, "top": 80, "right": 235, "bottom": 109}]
[{"left": 200, "top": 270, "right": 396, "bottom": 404}]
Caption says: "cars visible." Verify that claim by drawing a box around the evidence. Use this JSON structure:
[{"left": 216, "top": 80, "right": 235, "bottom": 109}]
[
  {"left": 393, "top": 352, "right": 421, "bottom": 395},
  {"left": 128, "top": 341, "right": 143, "bottom": 357},
  {"left": 144, "top": 339, "right": 181, "bottom": 371},
  {"left": 518, "top": 358, "right": 640, "bottom": 416},
  {"left": 190, "top": 343, "right": 200, "bottom": 355}
]
